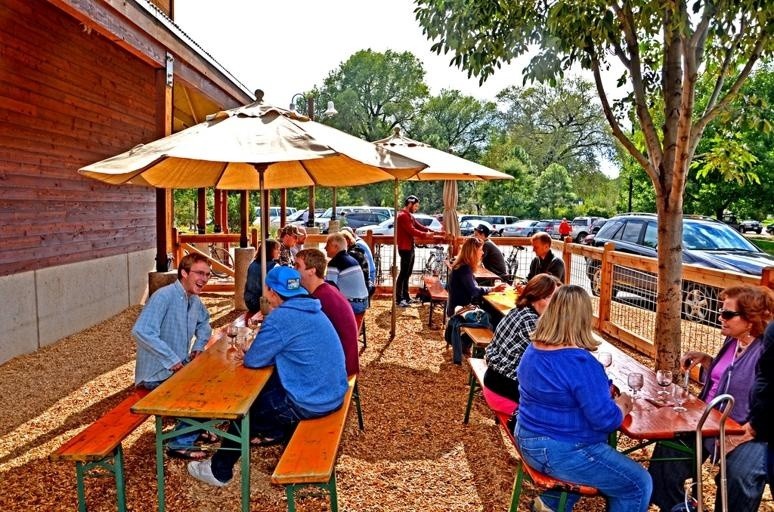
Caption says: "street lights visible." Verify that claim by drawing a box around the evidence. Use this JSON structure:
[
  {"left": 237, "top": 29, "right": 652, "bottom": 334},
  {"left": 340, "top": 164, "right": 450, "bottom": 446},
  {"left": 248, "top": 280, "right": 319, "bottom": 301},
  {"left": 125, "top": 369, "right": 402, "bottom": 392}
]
[{"left": 284, "top": 89, "right": 339, "bottom": 251}]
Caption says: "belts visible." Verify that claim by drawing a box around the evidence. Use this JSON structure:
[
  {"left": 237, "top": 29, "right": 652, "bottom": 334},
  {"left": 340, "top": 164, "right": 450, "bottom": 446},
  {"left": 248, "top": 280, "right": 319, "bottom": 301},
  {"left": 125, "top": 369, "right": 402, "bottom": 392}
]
[{"left": 348, "top": 298, "right": 365, "bottom": 302}]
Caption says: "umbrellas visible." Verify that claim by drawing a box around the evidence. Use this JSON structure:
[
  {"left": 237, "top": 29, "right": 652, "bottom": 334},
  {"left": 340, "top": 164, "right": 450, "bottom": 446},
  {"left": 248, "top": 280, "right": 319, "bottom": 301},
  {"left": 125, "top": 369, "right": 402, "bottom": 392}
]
[
  {"left": 72, "top": 90, "right": 429, "bottom": 300},
  {"left": 367, "top": 125, "right": 518, "bottom": 327}
]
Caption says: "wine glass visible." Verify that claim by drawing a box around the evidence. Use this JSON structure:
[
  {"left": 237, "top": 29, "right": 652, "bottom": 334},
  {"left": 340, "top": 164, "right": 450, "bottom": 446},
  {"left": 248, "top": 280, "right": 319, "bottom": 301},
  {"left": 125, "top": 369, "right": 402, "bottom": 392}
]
[
  {"left": 656, "top": 369, "right": 673, "bottom": 394},
  {"left": 671, "top": 383, "right": 690, "bottom": 412},
  {"left": 226, "top": 317, "right": 258, "bottom": 353},
  {"left": 491, "top": 276, "right": 529, "bottom": 295},
  {"left": 599, "top": 351, "right": 612, "bottom": 373},
  {"left": 627, "top": 372, "right": 645, "bottom": 399}
]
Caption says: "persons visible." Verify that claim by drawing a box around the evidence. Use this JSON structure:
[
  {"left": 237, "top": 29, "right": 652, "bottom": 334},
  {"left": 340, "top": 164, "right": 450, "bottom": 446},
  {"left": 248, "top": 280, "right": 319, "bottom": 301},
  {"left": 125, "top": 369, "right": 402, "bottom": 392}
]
[
  {"left": 749, "top": 319, "right": 774, "bottom": 499},
  {"left": 245, "top": 220, "right": 377, "bottom": 376},
  {"left": 395, "top": 193, "right": 445, "bottom": 306},
  {"left": 132, "top": 252, "right": 221, "bottom": 461},
  {"left": 513, "top": 286, "right": 654, "bottom": 512},
  {"left": 557, "top": 218, "right": 573, "bottom": 240},
  {"left": 187, "top": 266, "right": 349, "bottom": 488},
  {"left": 647, "top": 283, "right": 774, "bottom": 512},
  {"left": 447, "top": 224, "right": 565, "bottom": 429}
]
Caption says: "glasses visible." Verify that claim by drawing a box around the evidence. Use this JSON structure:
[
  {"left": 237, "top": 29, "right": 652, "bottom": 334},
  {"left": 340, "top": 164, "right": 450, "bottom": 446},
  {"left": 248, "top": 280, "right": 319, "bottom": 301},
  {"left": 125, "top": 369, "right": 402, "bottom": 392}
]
[
  {"left": 189, "top": 271, "right": 210, "bottom": 275},
  {"left": 719, "top": 308, "right": 743, "bottom": 320}
]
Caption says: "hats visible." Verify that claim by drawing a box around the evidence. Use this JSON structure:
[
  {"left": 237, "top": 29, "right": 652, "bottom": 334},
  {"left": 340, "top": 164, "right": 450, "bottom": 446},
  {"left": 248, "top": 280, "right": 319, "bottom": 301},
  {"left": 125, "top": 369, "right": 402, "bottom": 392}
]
[
  {"left": 283, "top": 225, "right": 304, "bottom": 236},
  {"left": 475, "top": 224, "right": 489, "bottom": 235},
  {"left": 265, "top": 266, "right": 309, "bottom": 298}
]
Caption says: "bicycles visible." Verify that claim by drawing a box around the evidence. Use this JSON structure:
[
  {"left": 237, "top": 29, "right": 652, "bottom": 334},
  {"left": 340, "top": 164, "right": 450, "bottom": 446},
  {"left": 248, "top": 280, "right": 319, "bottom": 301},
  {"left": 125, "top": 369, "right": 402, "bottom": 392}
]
[
  {"left": 372, "top": 242, "right": 384, "bottom": 284},
  {"left": 167, "top": 229, "right": 235, "bottom": 278},
  {"left": 503, "top": 245, "right": 526, "bottom": 286},
  {"left": 424, "top": 245, "right": 450, "bottom": 290}
]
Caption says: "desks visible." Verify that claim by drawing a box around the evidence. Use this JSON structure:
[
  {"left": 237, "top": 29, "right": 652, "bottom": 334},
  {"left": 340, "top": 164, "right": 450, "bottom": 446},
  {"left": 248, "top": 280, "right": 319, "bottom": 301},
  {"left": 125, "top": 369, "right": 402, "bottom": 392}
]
[
  {"left": 442, "top": 259, "right": 499, "bottom": 281},
  {"left": 588, "top": 329, "right": 746, "bottom": 511},
  {"left": 129, "top": 324, "right": 276, "bottom": 511}
]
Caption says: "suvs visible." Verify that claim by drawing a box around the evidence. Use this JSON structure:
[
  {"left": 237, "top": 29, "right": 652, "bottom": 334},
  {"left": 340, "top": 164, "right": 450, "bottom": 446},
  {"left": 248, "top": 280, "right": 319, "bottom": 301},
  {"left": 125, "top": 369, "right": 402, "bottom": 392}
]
[{"left": 585, "top": 212, "right": 774, "bottom": 326}]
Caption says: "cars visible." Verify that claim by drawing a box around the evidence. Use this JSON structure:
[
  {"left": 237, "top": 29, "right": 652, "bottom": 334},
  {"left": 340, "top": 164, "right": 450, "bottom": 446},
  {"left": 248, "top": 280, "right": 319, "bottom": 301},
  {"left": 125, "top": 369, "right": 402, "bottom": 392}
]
[
  {"left": 720, "top": 214, "right": 763, "bottom": 235},
  {"left": 766, "top": 223, "right": 774, "bottom": 236},
  {"left": 248, "top": 203, "right": 608, "bottom": 245}
]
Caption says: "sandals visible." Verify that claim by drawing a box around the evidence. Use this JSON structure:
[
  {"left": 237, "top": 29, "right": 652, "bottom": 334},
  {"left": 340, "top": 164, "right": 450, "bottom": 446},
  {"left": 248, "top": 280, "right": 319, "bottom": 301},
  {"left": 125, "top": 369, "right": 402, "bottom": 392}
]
[
  {"left": 250, "top": 435, "right": 278, "bottom": 446},
  {"left": 198, "top": 432, "right": 219, "bottom": 443},
  {"left": 167, "top": 448, "right": 206, "bottom": 459}
]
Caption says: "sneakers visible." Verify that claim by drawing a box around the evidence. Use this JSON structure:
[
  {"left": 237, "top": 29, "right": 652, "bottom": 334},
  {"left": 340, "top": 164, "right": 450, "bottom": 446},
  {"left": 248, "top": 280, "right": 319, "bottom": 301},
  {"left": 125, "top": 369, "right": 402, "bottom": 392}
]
[
  {"left": 529, "top": 495, "right": 554, "bottom": 512},
  {"left": 187, "top": 457, "right": 233, "bottom": 485},
  {"left": 397, "top": 299, "right": 418, "bottom": 307}
]
